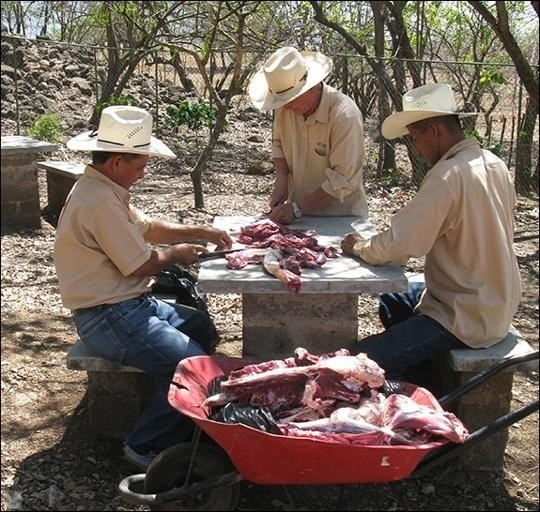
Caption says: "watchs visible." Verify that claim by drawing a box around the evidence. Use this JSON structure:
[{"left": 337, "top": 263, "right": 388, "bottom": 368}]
[{"left": 291, "top": 202, "right": 303, "bottom": 219}]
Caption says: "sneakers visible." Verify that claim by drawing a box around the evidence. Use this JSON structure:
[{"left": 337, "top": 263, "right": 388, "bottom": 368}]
[{"left": 124, "top": 437, "right": 159, "bottom": 470}]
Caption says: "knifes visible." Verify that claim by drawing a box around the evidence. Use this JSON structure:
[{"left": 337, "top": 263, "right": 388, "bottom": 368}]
[{"left": 192, "top": 249, "right": 248, "bottom": 263}]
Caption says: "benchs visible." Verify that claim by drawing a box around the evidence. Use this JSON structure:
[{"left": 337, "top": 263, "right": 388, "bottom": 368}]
[
  {"left": 432, "top": 324, "right": 539, "bottom": 470},
  {"left": 66, "top": 340, "right": 144, "bottom": 441},
  {"left": 38, "top": 162, "right": 85, "bottom": 212}
]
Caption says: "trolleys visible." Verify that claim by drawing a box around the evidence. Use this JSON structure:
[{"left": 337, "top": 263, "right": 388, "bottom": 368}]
[{"left": 164, "top": 344, "right": 540, "bottom": 511}]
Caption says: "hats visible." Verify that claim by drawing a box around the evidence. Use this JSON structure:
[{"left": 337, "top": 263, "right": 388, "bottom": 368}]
[
  {"left": 67, "top": 105, "right": 176, "bottom": 160},
  {"left": 381, "top": 84, "right": 478, "bottom": 139},
  {"left": 249, "top": 47, "right": 334, "bottom": 111}
]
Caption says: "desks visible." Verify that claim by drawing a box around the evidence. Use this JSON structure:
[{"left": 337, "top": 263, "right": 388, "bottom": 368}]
[
  {"left": 0, "top": 135, "right": 59, "bottom": 229},
  {"left": 198, "top": 213, "right": 408, "bottom": 360}
]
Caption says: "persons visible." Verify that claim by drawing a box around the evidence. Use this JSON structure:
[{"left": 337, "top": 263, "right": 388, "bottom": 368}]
[
  {"left": 246, "top": 47, "right": 371, "bottom": 226},
  {"left": 51, "top": 101, "right": 233, "bottom": 472},
  {"left": 338, "top": 81, "right": 523, "bottom": 387}
]
